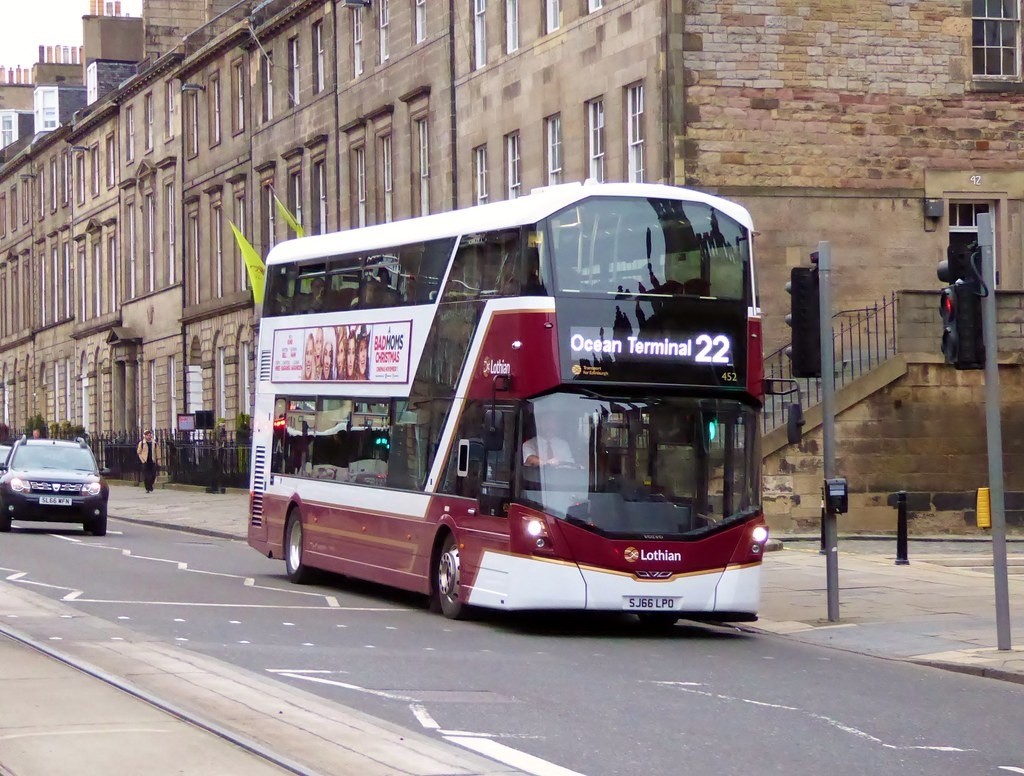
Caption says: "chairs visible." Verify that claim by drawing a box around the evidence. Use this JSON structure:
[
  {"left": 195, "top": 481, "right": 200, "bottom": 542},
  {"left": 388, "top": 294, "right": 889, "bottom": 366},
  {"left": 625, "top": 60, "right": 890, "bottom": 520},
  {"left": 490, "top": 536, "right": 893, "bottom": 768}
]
[
  {"left": 293, "top": 287, "right": 360, "bottom": 315},
  {"left": 592, "top": 278, "right": 712, "bottom": 296}
]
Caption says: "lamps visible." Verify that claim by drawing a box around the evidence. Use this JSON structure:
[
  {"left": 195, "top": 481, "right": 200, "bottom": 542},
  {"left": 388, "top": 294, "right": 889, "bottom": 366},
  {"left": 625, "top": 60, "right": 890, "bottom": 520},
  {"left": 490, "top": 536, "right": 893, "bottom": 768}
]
[
  {"left": 69, "top": 146, "right": 91, "bottom": 153},
  {"left": 183, "top": 82, "right": 206, "bottom": 95},
  {"left": 19, "top": 171, "right": 37, "bottom": 181}
]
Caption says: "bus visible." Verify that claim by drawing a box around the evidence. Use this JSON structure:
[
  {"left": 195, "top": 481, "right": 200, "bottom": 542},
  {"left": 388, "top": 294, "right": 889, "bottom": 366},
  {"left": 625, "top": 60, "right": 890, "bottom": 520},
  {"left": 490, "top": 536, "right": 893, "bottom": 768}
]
[{"left": 246, "top": 182, "right": 805, "bottom": 630}]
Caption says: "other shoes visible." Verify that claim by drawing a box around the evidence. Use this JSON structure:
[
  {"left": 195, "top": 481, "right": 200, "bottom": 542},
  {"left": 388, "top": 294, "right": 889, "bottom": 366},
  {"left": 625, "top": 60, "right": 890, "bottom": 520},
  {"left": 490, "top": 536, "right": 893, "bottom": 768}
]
[{"left": 146, "top": 488, "right": 153, "bottom": 493}]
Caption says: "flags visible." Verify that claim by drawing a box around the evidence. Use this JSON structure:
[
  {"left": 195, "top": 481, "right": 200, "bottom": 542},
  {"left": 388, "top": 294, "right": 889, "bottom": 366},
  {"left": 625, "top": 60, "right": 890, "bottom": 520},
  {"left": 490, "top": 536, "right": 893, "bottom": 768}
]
[
  {"left": 228, "top": 220, "right": 266, "bottom": 305},
  {"left": 273, "top": 194, "right": 305, "bottom": 239}
]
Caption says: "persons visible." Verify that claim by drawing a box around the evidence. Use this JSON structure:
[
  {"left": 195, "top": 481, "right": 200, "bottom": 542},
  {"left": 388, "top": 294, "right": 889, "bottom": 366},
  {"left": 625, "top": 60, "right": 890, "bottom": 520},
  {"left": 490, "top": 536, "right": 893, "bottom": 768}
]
[
  {"left": 137, "top": 430, "right": 161, "bottom": 492},
  {"left": 444, "top": 402, "right": 485, "bottom": 497},
  {"left": 317, "top": 430, "right": 348, "bottom": 466},
  {"left": 29, "top": 429, "right": 40, "bottom": 439},
  {"left": 524, "top": 412, "right": 584, "bottom": 469},
  {"left": 298, "top": 278, "right": 324, "bottom": 311},
  {"left": 352, "top": 253, "right": 522, "bottom": 309},
  {"left": 304, "top": 325, "right": 371, "bottom": 380}
]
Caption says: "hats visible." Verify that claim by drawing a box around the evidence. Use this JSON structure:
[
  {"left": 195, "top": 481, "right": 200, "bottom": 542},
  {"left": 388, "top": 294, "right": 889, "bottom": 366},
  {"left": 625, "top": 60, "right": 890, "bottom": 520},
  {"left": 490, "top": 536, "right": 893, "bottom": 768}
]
[{"left": 357, "top": 325, "right": 371, "bottom": 343}]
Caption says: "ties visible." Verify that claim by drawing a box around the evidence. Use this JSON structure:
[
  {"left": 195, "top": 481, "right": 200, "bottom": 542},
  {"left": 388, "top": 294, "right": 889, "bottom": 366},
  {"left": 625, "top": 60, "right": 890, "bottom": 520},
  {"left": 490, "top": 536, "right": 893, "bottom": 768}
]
[{"left": 546, "top": 441, "right": 553, "bottom": 460}]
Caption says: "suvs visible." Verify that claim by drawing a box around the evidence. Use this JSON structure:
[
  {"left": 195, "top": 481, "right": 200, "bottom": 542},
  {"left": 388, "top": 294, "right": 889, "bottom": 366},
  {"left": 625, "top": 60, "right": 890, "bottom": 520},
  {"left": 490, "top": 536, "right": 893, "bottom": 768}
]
[{"left": 0, "top": 435, "right": 113, "bottom": 537}]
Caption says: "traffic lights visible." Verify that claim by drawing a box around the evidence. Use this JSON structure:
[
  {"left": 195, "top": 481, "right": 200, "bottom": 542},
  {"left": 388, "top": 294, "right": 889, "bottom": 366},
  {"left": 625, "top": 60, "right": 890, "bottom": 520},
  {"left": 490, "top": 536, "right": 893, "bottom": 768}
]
[{"left": 939, "top": 286, "right": 984, "bottom": 370}]
